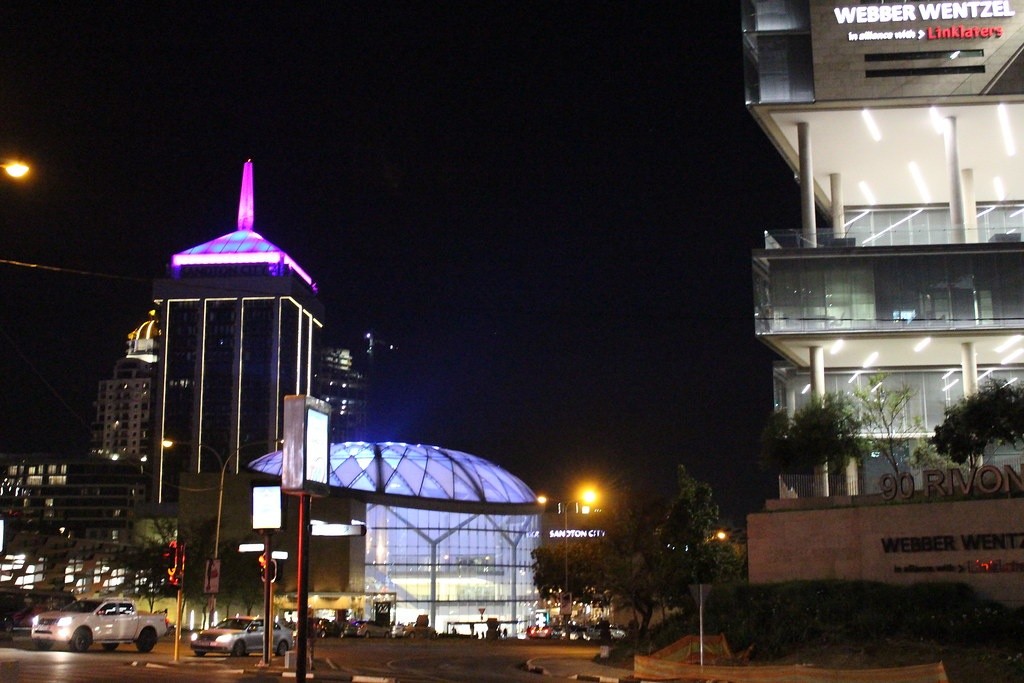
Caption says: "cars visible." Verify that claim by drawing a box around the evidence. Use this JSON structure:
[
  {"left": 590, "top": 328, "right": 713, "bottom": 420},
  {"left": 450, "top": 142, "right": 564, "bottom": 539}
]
[
  {"left": 307, "top": 618, "right": 438, "bottom": 640},
  {"left": 190, "top": 617, "right": 293, "bottom": 658},
  {"left": 528, "top": 624, "right": 625, "bottom": 641}
]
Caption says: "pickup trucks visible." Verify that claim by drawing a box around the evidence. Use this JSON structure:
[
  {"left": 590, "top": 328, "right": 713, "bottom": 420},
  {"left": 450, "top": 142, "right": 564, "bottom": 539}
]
[{"left": 31, "top": 599, "right": 168, "bottom": 653}]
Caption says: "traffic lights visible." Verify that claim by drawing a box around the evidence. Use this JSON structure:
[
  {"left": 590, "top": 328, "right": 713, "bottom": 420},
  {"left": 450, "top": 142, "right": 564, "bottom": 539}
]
[
  {"left": 163, "top": 547, "right": 173, "bottom": 568},
  {"left": 171, "top": 576, "right": 182, "bottom": 588}
]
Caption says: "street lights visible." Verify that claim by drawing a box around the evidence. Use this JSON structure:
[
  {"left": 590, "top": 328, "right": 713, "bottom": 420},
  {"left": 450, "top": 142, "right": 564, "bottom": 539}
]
[
  {"left": 564, "top": 493, "right": 594, "bottom": 640},
  {"left": 700, "top": 531, "right": 726, "bottom": 666},
  {"left": 163, "top": 438, "right": 286, "bottom": 624}
]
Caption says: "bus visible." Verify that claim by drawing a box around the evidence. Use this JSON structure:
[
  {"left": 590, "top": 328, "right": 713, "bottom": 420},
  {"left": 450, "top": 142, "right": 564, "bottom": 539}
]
[{"left": 0, "top": 586, "right": 79, "bottom": 633}]
